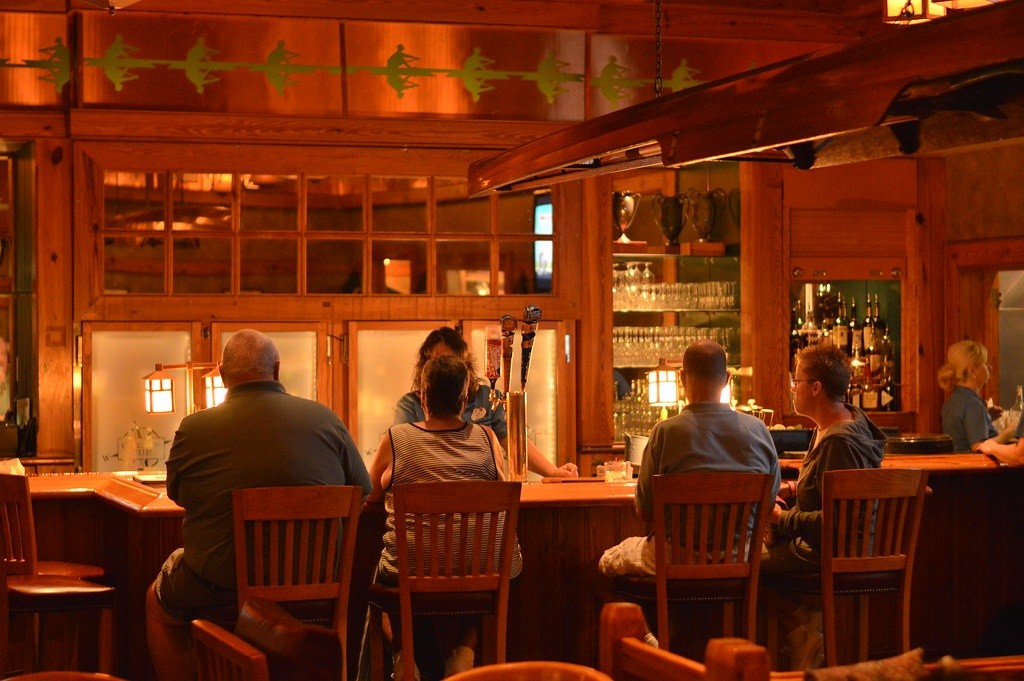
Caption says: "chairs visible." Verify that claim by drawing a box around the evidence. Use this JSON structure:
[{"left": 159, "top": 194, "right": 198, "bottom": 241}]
[
  {"left": 367, "top": 480, "right": 523, "bottom": 677},
  {"left": 193, "top": 481, "right": 365, "bottom": 681},
  {"left": 0, "top": 471, "right": 134, "bottom": 680},
  {"left": 764, "top": 468, "right": 935, "bottom": 669},
  {"left": 615, "top": 472, "right": 777, "bottom": 653}
]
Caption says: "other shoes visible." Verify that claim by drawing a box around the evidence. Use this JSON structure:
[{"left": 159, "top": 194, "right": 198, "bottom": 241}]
[
  {"left": 391, "top": 652, "right": 420, "bottom": 681},
  {"left": 445, "top": 646, "right": 475, "bottom": 679},
  {"left": 791, "top": 624, "right": 824, "bottom": 672}
]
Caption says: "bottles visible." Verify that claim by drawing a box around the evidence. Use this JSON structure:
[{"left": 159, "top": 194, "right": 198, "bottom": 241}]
[
  {"left": 1008, "top": 385, "right": 1024, "bottom": 429},
  {"left": 613, "top": 377, "right": 684, "bottom": 442},
  {"left": 791, "top": 283, "right": 896, "bottom": 413}
]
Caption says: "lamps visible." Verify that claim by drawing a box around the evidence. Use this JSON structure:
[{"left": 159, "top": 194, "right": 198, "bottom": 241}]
[{"left": 143, "top": 360, "right": 228, "bottom": 416}]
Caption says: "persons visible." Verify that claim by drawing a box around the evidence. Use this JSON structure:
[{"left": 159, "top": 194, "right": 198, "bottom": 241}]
[
  {"left": 975, "top": 411, "right": 1024, "bottom": 467},
  {"left": 768, "top": 342, "right": 888, "bottom": 669},
  {"left": 393, "top": 327, "right": 580, "bottom": 478},
  {"left": 938, "top": 339, "right": 1018, "bottom": 455},
  {"left": 583, "top": 339, "right": 782, "bottom": 650},
  {"left": 145, "top": 328, "right": 373, "bottom": 681},
  {"left": 365, "top": 355, "right": 524, "bottom": 681}
]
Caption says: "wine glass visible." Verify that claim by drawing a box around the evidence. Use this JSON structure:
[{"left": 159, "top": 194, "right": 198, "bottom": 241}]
[
  {"left": 613, "top": 260, "right": 655, "bottom": 285},
  {"left": 613, "top": 325, "right": 738, "bottom": 365}
]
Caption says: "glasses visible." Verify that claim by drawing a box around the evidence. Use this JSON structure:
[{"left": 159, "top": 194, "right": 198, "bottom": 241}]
[{"left": 791, "top": 378, "right": 815, "bottom": 389}]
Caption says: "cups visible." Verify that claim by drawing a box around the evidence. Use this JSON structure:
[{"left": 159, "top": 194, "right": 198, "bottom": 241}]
[
  {"left": 603, "top": 460, "right": 626, "bottom": 484},
  {"left": 613, "top": 281, "right": 740, "bottom": 310}
]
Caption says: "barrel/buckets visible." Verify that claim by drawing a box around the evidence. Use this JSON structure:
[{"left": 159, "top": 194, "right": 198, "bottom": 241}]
[{"left": 621, "top": 431, "right": 649, "bottom": 478}]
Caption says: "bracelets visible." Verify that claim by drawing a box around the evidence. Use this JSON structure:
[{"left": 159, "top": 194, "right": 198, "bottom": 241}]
[{"left": 788, "top": 481, "right": 797, "bottom": 498}]
